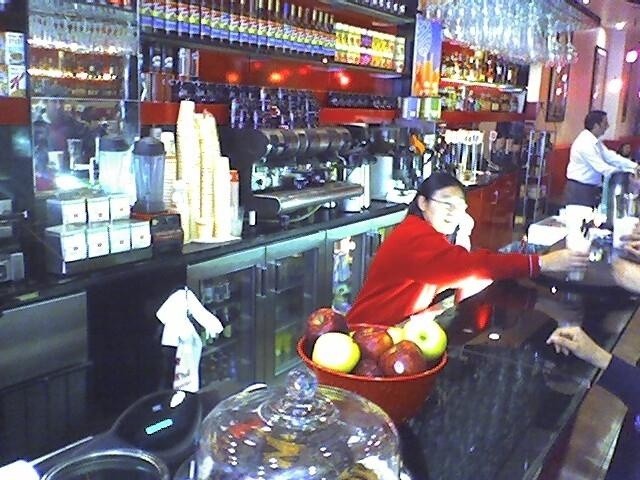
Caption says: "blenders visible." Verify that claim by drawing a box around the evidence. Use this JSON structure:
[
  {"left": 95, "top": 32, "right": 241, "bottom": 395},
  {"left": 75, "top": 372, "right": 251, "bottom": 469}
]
[{"left": 132, "top": 136, "right": 183, "bottom": 247}]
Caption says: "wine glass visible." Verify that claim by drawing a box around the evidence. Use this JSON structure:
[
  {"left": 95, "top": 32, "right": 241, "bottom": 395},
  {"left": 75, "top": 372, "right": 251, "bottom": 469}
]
[{"left": 433, "top": 0, "right": 580, "bottom": 68}]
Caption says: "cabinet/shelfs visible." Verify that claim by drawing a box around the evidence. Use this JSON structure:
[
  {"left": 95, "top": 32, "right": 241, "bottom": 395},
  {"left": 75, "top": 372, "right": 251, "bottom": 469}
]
[
  {"left": 519, "top": 129, "right": 555, "bottom": 231},
  {"left": 0, "top": 1, "right": 30, "bottom": 127},
  {"left": 461, "top": 182, "right": 483, "bottom": 250},
  {"left": 442, "top": 42, "right": 531, "bottom": 128},
  {"left": 191, "top": 229, "right": 328, "bottom": 392},
  {"left": 140, "top": 0, "right": 414, "bottom": 127},
  {"left": 328, "top": 209, "right": 410, "bottom": 321},
  {"left": 481, "top": 169, "right": 524, "bottom": 254}
]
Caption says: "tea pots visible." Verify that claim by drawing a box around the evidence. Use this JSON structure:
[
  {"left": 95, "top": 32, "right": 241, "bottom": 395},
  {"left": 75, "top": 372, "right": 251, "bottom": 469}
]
[{"left": 89, "top": 134, "right": 132, "bottom": 195}]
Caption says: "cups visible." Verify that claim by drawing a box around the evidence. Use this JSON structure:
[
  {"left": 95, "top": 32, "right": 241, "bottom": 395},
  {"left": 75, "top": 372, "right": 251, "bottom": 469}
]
[
  {"left": 566, "top": 240, "right": 591, "bottom": 282},
  {"left": 163, "top": 101, "right": 243, "bottom": 247}
]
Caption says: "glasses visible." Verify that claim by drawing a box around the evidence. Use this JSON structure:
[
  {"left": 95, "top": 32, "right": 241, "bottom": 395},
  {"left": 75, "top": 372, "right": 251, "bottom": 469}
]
[{"left": 428, "top": 195, "right": 469, "bottom": 214}]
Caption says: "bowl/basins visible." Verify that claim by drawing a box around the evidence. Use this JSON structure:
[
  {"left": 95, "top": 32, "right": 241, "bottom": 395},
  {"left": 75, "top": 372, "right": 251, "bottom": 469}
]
[{"left": 296, "top": 323, "right": 448, "bottom": 424}]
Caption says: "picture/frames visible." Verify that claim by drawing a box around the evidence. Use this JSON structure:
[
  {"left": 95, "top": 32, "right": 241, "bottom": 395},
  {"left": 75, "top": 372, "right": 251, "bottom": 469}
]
[
  {"left": 589, "top": 45, "right": 610, "bottom": 111},
  {"left": 548, "top": 27, "right": 574, "bottom": 123}
]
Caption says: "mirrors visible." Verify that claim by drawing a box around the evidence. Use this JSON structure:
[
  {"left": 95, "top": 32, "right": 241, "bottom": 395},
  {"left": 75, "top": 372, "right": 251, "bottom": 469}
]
[{"left": 25, "top": 0, "right": 138, "bottom": 196}]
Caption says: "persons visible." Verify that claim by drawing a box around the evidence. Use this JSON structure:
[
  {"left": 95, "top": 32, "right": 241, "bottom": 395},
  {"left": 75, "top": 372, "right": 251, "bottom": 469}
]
[
  {"left": 562, "top": 108, "right": 640, "bottom": 207},
  {"left": 612, "top": 140, "right": 637, "bottom": 164},
  {"left": 351, "top": 170, "right": 592, "bottom": 340},
  {"left": 540, "top": 322, "right": 639, "bottom": 413}
]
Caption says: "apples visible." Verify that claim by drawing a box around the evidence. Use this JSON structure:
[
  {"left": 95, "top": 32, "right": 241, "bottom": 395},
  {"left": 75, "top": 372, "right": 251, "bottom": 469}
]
[
  {"left": 405, "top": 315, "right": 447, "bottom": 362},
  {"left": 353, "top": 325, "right": 393, "bottom": 362},
  {"left": 312, "top": 332, "right": 360, "bottom": 374},
  {"left": 306, "top": 307, "right": 350, "bottom": 349},
  {"left": 387, "top": 326, "right": 408, "bottom": 344},
  {"left": 353, "top": 358, "right": 383, "bottom": 377},
  {"left": 379, "top": 340, "right": 427, "bottom": 377}
]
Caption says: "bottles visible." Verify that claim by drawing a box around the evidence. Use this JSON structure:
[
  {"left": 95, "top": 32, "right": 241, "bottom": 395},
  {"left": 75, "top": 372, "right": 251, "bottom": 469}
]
[
  {"left": 440, "top": 68, "right": 525, "bottom": 114},
  {"left": 138, "top": 0, "right": 336, "bottom": 64}
]
